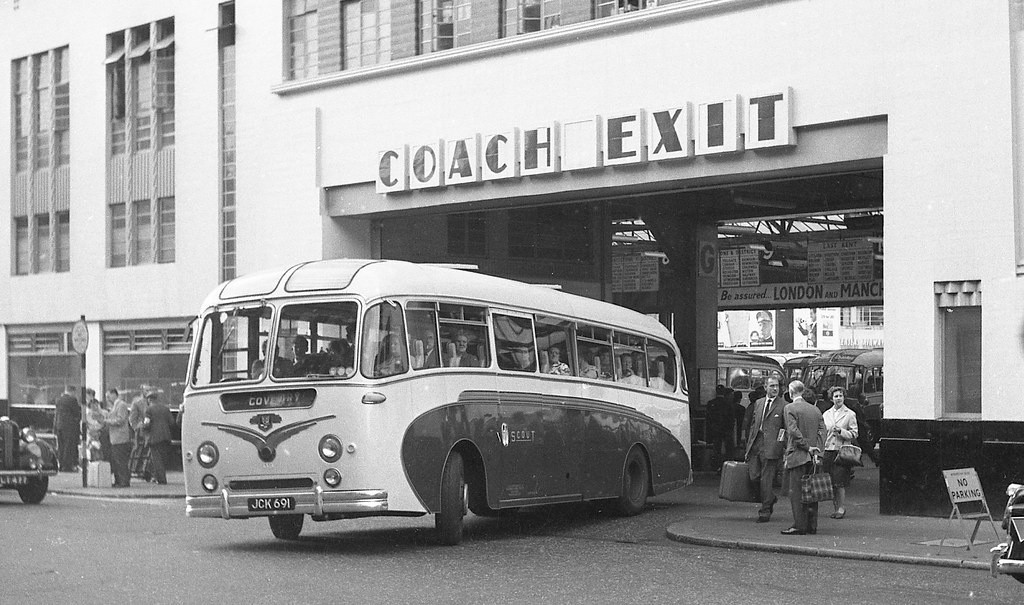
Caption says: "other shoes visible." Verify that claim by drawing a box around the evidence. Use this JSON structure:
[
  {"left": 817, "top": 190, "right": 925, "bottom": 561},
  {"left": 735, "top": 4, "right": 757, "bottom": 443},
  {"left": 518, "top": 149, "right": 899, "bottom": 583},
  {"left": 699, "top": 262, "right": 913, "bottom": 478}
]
[
  {"left": 756, "top": 507, "right": 773, "bottom": 523},
  {"left": 112, "top": 484, "right": 130, "bottom": 488},
  {"left": 831, "top": 508, "right": 846, "bottom": 519}
]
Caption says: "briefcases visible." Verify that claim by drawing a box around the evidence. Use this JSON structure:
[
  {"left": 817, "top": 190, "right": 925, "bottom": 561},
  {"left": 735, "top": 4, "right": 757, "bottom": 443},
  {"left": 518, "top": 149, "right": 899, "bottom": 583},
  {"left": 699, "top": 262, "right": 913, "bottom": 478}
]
[
  {"left": 719, "top": 462, "right": 759, "bottom": 502},
  {"left": 86, "top": 460, "right": 112, "bottom": 487}
]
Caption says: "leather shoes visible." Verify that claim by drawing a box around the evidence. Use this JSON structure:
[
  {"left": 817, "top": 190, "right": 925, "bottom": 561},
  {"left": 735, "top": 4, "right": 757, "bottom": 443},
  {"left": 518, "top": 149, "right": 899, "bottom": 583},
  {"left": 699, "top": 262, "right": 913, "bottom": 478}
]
[{"left": 781, "top": 527, "right": 816, "bottom": 535}]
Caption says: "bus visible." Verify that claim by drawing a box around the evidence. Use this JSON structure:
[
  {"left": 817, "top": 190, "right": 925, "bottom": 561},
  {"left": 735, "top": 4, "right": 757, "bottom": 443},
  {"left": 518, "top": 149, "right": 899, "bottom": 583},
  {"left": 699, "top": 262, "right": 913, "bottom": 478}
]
[
  {"left": 717, "top": 349, "right": 883, "bottom": 406},
  {"left": 178, "top": 258, "right": 693, "bottom": 544}
]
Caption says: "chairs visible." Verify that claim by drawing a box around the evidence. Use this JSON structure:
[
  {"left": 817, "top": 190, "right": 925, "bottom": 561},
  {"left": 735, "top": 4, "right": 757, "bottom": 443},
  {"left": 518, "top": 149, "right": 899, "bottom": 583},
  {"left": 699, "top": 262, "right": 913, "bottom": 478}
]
[
  {"left": 615, "top": 357, "right": 623, "bottom": 380},
  {"left": 476, "top": 344, "right": 487, "bottom": 367},
  {"left": 412, "top": 340, "right": 425, "bottom": 369},
  {"left": 658, "top": 362, "right": 667, "bottom": 380},
  {"left": 594, "top": 355, "right": 602, "bottom": 375},
  {"left": 447, "top": 342, "right": 461, "bottom": 367},
  {"left": 636, "top": 359, "right": 644, "bottom": 378},
  {"left": 540, "top": 350, "right": 550, "bottom": 373}
]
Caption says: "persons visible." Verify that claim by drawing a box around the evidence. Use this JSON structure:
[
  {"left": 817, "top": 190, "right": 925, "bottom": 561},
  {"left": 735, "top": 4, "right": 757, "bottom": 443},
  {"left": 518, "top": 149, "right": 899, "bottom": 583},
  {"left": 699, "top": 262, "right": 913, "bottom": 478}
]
[
  {"left": 796, "top": 310, "right": 818, "bottom": 348},
  {"left": 745, "top": 376, "right": 790, "bottom": 523},
  {"left": 78, "top": 384, "right": 181, "bottom": 488},
  {"left": 750, "top": 310, "right": 774, "bottom": 347},
  {"left": 781, "top": 380, "right": 827, "bottom": 536},
  {"left": 54, "top": 385, "right": 81, "bottom": 473},
  {"left": 252, "top": 321, "right": 646, "bottom": 379},
  {"left": 822, "top": 386, "right": 858, "bottom": 519},
  {"left": 706, "top": 385, "right": 883, "bottom": 474}
]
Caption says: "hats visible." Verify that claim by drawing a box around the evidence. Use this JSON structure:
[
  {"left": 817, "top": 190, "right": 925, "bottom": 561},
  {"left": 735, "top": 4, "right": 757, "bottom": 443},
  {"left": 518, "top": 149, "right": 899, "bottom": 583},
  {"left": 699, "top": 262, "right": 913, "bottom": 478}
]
[{"left": 756, "top": 312, "right": 772, "bottom": 323}]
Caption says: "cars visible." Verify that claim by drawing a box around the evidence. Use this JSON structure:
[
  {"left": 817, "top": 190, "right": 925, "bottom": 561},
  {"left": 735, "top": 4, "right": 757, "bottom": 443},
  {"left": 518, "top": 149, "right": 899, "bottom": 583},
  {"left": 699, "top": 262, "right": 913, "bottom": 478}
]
[
  {"left": 988, "top": 484, "right": 1024, "bottom": 582},
  {"left": 125, "top": 408, "right": 183, "bottom": 470},
  {"left": 0, "top": 415, "right": 57, "bottom": 503}
]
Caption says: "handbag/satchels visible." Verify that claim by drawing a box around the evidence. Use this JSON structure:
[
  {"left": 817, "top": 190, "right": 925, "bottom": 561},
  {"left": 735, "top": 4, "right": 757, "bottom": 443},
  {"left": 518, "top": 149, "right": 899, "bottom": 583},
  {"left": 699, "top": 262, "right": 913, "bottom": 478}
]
[
  {"left": 800, "top": 463, "right": 834, "bottom": 505},
  {"left": 833, "top": 431, "right": 864, "bottom": 467}
]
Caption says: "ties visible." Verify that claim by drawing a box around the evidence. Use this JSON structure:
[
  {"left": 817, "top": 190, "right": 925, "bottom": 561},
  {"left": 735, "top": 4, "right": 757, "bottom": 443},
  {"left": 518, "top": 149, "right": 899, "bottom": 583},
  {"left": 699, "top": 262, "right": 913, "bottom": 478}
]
[{"left": 764, "top": 400, "right": 772, "bottom": 415}]
